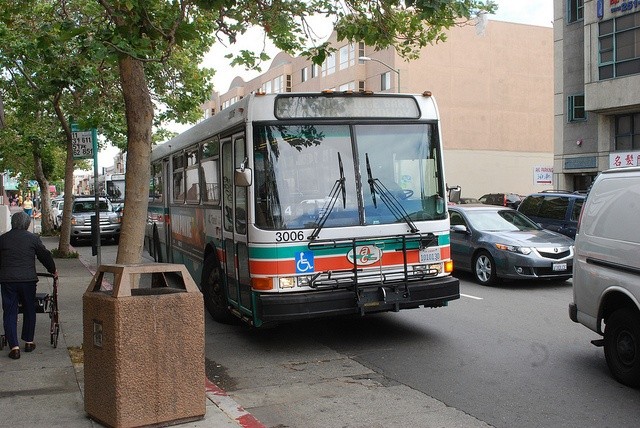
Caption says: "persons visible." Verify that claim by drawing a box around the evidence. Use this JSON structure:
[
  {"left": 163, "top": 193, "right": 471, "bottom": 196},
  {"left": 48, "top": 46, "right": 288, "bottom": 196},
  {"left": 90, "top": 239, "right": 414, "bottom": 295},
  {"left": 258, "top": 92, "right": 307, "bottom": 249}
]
[
  {"left": 9, "top": 193, "right": 23, "bottom": 207},
  {"left": 1, "top": 213, "right": 57, "bottom": 360},
  {"left": 23, "top": 197, "right": 34, "bottom": 220}
]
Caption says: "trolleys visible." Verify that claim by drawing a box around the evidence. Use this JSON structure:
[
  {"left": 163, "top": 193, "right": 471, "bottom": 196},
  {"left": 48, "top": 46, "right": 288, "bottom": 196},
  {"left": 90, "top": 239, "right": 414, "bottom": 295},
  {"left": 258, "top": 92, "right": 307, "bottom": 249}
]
[{"left": 0, "top": 270, "right": 61, "bottom": 351}]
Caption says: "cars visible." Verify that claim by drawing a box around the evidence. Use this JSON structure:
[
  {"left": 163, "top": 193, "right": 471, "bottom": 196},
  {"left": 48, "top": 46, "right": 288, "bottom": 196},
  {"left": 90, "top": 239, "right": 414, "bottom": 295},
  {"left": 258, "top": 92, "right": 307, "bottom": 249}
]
[
  {"left": 458, "top": 197, "right": 480, "bottom": 203},
  {"left": 449, "top": 202, "right": 575, "bottom": 286},
  {"left": 47, "top": 197, "right": 64, "bottom": 229}
]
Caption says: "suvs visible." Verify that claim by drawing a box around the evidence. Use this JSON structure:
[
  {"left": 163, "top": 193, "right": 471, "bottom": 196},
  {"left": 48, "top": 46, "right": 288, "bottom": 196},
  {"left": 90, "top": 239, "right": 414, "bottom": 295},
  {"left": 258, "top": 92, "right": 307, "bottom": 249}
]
[
  {"left": 515, "top": 189, "right": 586, "bottom": 236},
  {"left": 65, "top": 195, "right": 121, "bottom": 244}
]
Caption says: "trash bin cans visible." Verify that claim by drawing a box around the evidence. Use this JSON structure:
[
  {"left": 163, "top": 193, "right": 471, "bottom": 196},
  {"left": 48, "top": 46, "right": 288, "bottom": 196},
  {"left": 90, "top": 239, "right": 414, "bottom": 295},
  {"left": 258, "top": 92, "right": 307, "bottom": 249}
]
[{"left": 83, "top": 262, "right": 205, "bottom": 428}]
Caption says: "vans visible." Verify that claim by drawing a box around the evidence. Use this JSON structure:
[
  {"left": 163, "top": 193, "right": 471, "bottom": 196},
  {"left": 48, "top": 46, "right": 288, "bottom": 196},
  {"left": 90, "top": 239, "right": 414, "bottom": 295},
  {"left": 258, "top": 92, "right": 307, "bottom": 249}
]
[
  {"left": 478, "top": 192, "right": 520, "bottom": 207},
  {"left": 568, "top": 167, "right": 640, "bottom": 386}
]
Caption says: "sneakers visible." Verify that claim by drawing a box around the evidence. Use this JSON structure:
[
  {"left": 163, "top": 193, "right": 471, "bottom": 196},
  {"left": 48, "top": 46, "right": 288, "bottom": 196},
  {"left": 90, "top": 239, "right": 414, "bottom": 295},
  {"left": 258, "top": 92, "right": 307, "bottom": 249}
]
[
  {"left": 8, "top": 349, "right": 20, "bottom": 359},
  {"left": 25, "top": 343, "right": 35, "bottom": 352}
]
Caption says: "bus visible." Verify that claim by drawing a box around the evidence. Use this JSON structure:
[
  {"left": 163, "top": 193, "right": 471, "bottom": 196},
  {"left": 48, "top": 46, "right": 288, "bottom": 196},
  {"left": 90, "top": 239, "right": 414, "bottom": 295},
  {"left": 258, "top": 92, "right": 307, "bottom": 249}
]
[
  {"left": 89, "top": 173, "right": 125, "bottom": 213},
  {"left": 143, "top": 88, "right": 461, "bottom": 329}
]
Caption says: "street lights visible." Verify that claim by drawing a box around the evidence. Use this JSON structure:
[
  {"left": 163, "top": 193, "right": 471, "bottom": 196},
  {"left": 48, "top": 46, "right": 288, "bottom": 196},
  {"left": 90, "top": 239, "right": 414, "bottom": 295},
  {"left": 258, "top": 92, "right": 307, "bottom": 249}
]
[{"left": 356, "top": 55, "right": 405, "bottom": 93}]
